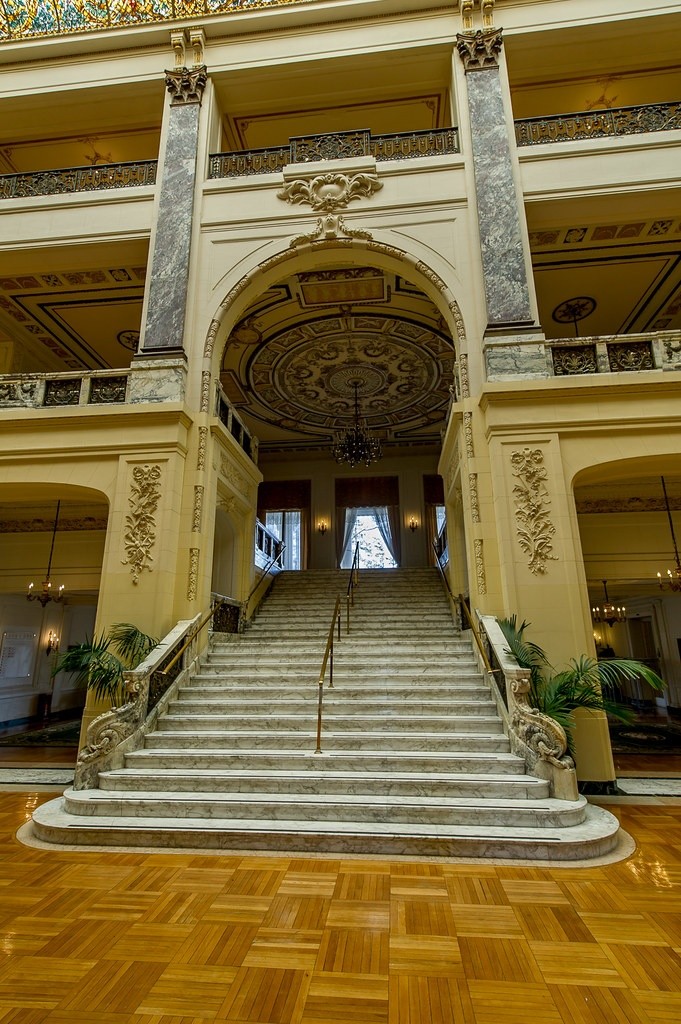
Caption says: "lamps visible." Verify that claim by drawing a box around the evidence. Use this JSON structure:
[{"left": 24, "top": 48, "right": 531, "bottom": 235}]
[
  {"left": 331, "top": 378, "right": 385, "bottom": 468},
  {"left": 587, "top": 579, "right": 627, "bottom": 628},
  {"left": 657, "top": 476, "right": 681, "bottom": 595},
  {"left": 408, "top": 516, "right": 421, "bottom": 532},
  {"left": 318, "top": 521, "right": 328, "bottom": 537},
  {"left": 46, "top": 631, "right": 60, "bottom": 658},
  {"left": 592, "top": 632, "right": 602, "bottom": 649},
  {"left": 26, "top": 499, "right": 66, "bottom": 609}
]
